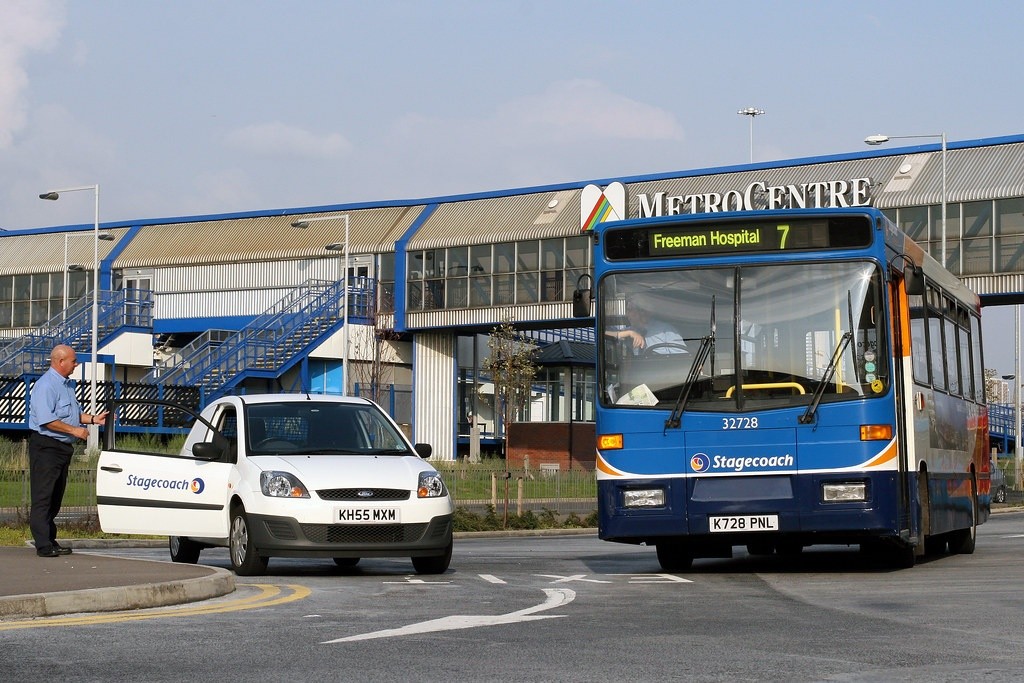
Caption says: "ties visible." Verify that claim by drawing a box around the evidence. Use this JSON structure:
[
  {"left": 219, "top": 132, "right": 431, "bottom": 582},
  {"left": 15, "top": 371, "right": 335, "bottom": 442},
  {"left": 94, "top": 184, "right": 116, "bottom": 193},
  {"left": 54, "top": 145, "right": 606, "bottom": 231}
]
[{"left": 637, "top": 328, "right": 647, "bottom": 357}]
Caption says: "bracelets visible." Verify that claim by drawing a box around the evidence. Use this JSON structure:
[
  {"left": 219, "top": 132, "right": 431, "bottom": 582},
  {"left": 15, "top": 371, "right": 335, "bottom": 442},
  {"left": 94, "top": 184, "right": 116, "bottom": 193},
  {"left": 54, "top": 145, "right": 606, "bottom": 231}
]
[{"left": 91, "top": 415, "right": 95, "bottom": 426}]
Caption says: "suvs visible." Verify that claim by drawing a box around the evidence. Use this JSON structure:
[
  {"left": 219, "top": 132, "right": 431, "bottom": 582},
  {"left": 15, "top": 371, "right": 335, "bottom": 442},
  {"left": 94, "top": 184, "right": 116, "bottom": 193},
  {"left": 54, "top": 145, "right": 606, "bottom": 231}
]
[{"left": 96, "top": 390, "right": 456, "bottom": 579}]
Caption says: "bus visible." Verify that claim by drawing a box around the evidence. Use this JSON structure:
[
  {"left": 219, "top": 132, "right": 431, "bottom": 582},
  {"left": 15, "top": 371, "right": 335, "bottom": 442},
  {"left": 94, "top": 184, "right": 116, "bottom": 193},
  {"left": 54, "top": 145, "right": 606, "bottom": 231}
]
[{"left": 574, "top": 204, "right": 992, "bottom": 572}]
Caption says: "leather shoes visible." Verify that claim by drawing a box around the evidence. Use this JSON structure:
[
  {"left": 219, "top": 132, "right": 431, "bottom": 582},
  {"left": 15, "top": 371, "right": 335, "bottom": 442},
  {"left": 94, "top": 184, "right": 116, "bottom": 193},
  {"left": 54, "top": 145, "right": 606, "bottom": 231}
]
[
  {"left": 37, "top": 548, "right": 59, "bottom": 557},
  {"left": 53, "top": 542, "right": 72, "bottom": 555}
]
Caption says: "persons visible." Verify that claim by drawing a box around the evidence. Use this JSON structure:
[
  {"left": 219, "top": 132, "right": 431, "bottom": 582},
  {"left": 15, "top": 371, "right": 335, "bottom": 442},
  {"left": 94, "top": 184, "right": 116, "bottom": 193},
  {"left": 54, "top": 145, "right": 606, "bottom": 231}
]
[
  {"left": 605, "top": 296, "right": 691, "bottom": 354},
  {"left": 28, "top": 345, "right": 117, "bottom": 557}
]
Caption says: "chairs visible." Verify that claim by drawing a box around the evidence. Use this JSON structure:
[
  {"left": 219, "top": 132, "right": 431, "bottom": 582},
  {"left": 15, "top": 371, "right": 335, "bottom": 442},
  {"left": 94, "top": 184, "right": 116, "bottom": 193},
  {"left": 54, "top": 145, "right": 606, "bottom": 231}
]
[{"left": 249, "top": 418, "right": 266, "bottom": 441}]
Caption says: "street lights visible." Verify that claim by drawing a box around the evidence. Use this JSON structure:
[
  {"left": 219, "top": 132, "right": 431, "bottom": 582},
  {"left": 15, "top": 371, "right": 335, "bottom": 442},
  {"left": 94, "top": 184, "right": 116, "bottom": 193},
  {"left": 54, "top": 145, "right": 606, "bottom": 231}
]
[
  {"left": 866, "top": 132, "right": 947, "bottom": 271},
  {"left": 291, "top": 213, "right": 349, "bottom": 398},
  {"left": 738, "top": 108, "right": 765, "bottom": 163},
  {"left": 62, "top": 231, "right": 115, "bottom": 323},
  {"left": 39, "top": 183, "right": 100, "bottom": 453}
]
